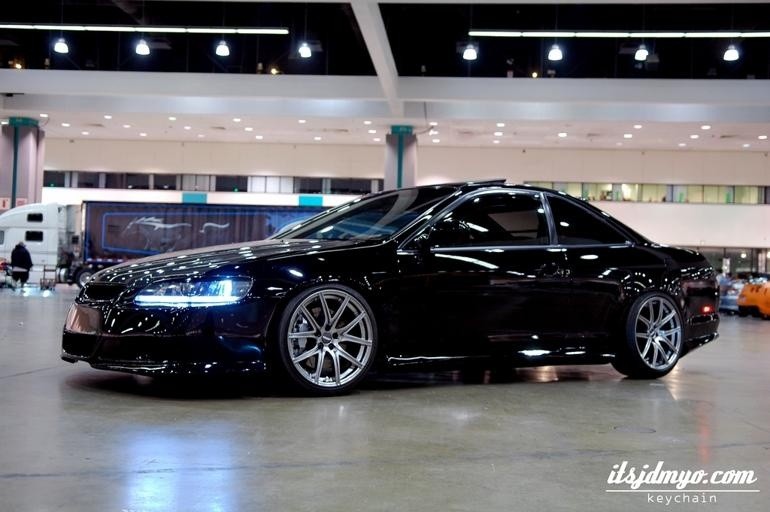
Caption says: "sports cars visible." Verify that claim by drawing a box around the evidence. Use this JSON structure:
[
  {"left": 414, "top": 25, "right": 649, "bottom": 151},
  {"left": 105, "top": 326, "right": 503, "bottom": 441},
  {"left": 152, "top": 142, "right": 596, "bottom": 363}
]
[{"left": 54, "top": 172, "right": 723, "bottom": 397}]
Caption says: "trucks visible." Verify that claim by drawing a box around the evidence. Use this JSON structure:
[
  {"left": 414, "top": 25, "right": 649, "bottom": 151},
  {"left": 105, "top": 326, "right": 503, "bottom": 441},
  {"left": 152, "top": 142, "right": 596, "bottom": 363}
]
[{"left": 1, "top": 198, "right": 367, "bottom": 293}]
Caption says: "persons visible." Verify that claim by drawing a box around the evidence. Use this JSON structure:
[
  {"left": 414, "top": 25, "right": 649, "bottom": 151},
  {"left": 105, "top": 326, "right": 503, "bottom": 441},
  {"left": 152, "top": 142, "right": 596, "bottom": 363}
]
[{"left": 11, "top": 242, "right": 32, "bottom": 287}]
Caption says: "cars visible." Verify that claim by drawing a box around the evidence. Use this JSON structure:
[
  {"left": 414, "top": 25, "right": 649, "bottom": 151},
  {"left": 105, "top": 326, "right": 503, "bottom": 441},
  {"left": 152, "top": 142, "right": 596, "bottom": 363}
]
[{"left": 715, "top": 269, "right": 770, "bottom": 322}]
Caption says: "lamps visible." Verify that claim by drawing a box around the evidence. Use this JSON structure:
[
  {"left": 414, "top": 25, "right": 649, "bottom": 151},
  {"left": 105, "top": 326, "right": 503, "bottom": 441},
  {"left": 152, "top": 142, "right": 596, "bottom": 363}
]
[
  {"left": 8, "top": 20, "right": 315, "bottom": 61},
  {"left": 462, "top": 30, "right": 770, "bottom": 64}
]
